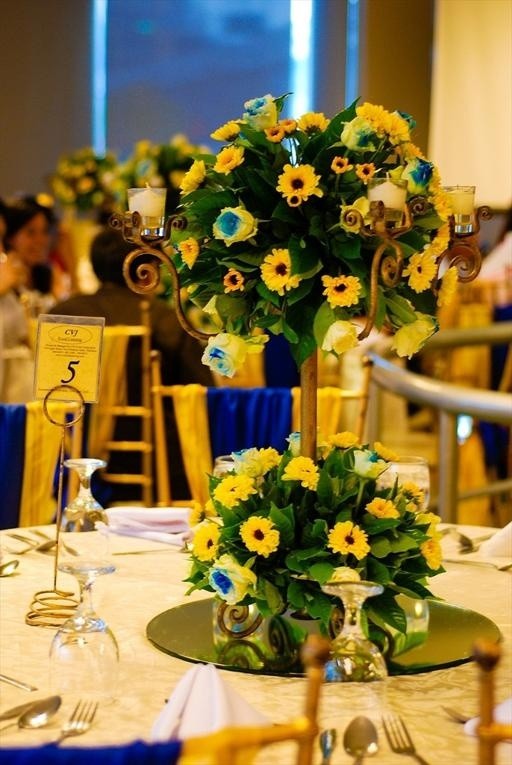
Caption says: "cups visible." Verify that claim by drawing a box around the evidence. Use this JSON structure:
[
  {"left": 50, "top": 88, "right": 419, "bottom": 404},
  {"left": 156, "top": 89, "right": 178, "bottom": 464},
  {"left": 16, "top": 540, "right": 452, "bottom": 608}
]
[
  {"left": 438, "top": 185, "right": 476, "bottom": 234},
  {"left": 367, "top": 178, "right": 408, "bottom": 222},
  {"left": 384, "top": 455, "right": 431, "bottom": 514},
  {"left": 127, "top": 187, "right": 167, "bottom": 237}
]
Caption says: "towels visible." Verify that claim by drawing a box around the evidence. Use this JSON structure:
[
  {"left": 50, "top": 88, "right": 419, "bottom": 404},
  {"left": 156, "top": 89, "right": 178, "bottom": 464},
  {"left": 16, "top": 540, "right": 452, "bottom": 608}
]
[
  {"left": 94, "top": 505, "right": 196, "bottom": 547},
  {"left": 147, "top": 662, "right": 271, "bottom": 741},
  {"left": 460, "top": 695, "right": 512, "bottom": 741},
  {"left": 479, "top": 520, "right": 512, "bottom": 558},
  {"left": 169, "top": 381, "right": 343, "bottom": 503},
  {"left": 0, "top": 402, "right": 96, "bottom": 528}
]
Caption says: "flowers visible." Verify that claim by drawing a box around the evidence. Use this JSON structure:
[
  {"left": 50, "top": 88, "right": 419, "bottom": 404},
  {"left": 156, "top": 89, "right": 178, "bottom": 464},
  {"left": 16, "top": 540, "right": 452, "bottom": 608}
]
[{"left": 105, "top": 91, "right": 485, "bottom": 684}]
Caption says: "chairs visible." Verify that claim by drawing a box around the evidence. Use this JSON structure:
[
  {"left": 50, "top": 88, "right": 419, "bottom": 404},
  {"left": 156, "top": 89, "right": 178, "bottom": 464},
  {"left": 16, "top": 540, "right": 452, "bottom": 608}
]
[{"left": 24, "top": 320, "right": 155, "bottom": 514}]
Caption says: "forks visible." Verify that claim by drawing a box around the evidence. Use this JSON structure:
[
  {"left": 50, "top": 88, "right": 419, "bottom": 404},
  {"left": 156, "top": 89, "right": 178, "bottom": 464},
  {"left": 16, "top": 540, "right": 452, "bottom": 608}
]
[
  {"left": 46, "top": 700, "right": 99, "bottom": 747},
  {"left": 0, "top": 543, "right": 32, "bottom": 555},
  {"left": 381, "top": 714, "right": 430, "bottom": 765}
]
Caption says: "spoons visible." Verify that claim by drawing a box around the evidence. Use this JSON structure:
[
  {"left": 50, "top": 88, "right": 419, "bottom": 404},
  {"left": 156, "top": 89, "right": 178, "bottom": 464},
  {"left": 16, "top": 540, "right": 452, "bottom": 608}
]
[
  {"left": 343, "top": 716, "right": 379, "bottom": 765},
  {"left": 8, "top": 531, "right": 57, "bottom": 551},
  {"left": 0, "top": 560, "right": 19, "bottom": 577},
  {"left": 450, "top": 527, "right": 499, "bottom": 548},
  {"left": 441, "top": 556, "right": 512, "bottom": 571},
  {"left": 0, "top": 695, "right": 62, "bottom": 732}
]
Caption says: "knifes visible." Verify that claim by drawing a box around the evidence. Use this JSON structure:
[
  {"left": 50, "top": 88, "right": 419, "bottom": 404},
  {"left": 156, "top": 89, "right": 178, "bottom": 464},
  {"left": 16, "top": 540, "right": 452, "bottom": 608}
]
[
  {"left": 0, "top": 700, "right": 45, "bottom": 721},
  {"left": 31, "top": 529, "right": 78, "bottom": 556},
  {"left": 319, "top": 728, "right": 336, "bottom": 765}
]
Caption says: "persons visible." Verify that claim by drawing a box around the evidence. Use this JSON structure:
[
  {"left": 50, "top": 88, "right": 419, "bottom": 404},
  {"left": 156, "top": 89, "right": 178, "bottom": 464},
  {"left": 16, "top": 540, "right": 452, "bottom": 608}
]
[
  {"left": 2, "top": 200, "right": 55, "bottom": 292},
  {"left": 0, "top": 197, "right": 28, "bottom": 402},
  {"left": 43, "top": 227, "right": 212, "bottom": 502}
]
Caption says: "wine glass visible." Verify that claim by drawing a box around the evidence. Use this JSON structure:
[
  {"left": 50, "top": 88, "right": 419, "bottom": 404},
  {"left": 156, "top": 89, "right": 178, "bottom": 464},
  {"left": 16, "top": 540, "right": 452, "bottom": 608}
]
[
  {"left": 48, "top": 560, "right": 121, "bottom": 708},
  {"left": 59, "top": 457, "right": 110, "bottom": 560},
  {"left": 317, "top": 581, "right": 390, "bottom": 737}
]
